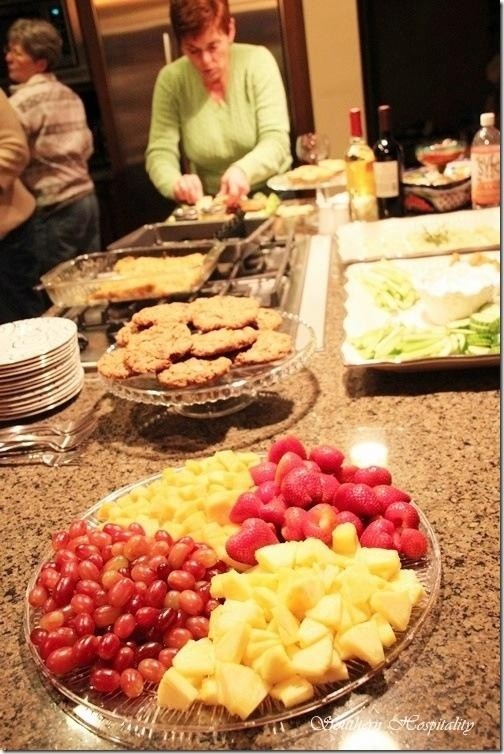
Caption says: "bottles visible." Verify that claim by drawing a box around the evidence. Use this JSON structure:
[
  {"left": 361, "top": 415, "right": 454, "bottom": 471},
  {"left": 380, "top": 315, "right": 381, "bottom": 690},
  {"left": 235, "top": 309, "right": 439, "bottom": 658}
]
[
  {"left": 374, "top": 104, "right": 405, "bottom": 219},
  {"left": 345, "top": 107, "right": 379, "bottom": 223},
  {"left": 470, "top": 111, "right": 501, "bottom": 209}
]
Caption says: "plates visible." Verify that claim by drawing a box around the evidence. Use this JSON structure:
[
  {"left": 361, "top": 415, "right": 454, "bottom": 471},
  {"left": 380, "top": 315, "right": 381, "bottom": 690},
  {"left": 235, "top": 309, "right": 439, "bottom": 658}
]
[
  {"left": 21, "top": 451, "right": 445, "bottom": 734},
  {"left": 0, "top": 316, "right": 84, "bottom": 423}
]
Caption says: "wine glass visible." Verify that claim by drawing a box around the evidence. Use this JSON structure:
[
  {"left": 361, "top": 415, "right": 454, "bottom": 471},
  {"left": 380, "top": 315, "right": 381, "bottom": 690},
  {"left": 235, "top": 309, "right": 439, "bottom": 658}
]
[
  {"left": 414, "top": 140, "right": 466, "bottom": 186},
  {"left": 296, "top": 132, "right": 330, "bottom": 166}
]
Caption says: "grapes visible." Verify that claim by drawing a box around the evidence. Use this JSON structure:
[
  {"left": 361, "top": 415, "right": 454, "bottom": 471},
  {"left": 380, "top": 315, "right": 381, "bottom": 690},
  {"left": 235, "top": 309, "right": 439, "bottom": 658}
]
[{"left": 29, "top": 519, "right": 228, "bottom": 699}]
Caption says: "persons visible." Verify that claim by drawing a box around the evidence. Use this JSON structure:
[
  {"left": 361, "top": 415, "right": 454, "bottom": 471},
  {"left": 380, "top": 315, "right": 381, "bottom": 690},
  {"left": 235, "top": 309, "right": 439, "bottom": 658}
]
[
  {"left": 142, "top": 0, "right": 297, "bottom": 205},
  {"left": 1, "top": 86, "right": 42, "bottom": 326},
  {"left": 0, "top": 14, "right": 101, "bottom": 274}
]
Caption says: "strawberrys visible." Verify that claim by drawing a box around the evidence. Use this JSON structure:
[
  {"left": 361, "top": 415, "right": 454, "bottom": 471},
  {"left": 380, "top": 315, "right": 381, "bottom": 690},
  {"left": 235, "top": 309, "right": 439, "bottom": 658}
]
[{"left": 225, "top": 435, "right": 429, "bottom": 568}]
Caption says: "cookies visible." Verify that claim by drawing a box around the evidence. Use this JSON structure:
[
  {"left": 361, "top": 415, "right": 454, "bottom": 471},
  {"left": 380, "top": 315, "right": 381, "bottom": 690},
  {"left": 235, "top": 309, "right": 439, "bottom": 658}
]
[{"left": 96, "top": 294, "right": 295, "bottom": 389}]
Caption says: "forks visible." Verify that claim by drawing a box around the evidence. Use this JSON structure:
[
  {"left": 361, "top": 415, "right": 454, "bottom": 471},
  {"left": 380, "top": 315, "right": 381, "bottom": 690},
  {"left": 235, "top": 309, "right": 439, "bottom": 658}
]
[{"left": 0, "top": 417, "right": 98, "bottom": 467}]
